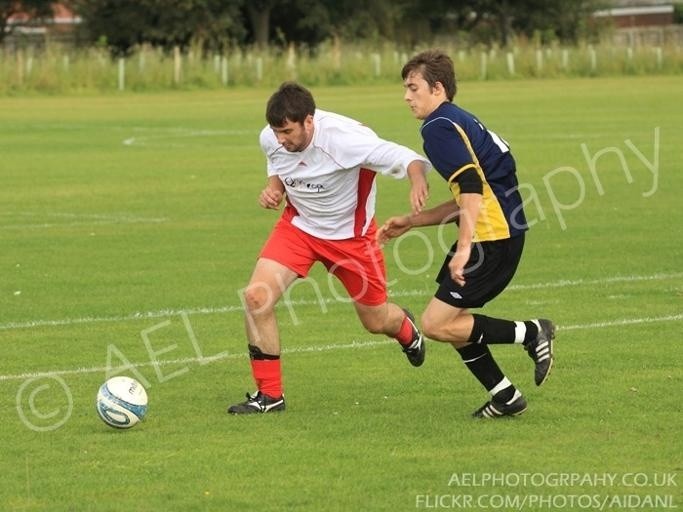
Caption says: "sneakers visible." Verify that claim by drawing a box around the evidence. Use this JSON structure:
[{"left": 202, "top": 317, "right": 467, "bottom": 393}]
[
  {"left": 401, "top": 306, "right": 426, "bottom": 367},
  {"left": 524, "top": 317, "right": 557, "bottom": 389},
  {"left": 226, "top": 390, "right": 286, "bottom": 415},
  {"left": 470, "top": 387, "right": 529, "bottom": 420}
]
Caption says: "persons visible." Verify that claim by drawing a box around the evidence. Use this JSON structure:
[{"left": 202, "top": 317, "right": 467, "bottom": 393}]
[
  {"left": 372, "top": 47, "right": 555, "bottom": 418},
  {"left": 227, "top": 82, "right": 433, "bottom": 414}
]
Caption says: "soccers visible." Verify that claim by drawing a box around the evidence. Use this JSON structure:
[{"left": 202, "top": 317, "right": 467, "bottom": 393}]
[{"left": 97, "top": 377, "right": 148, "bottom": 428}]
